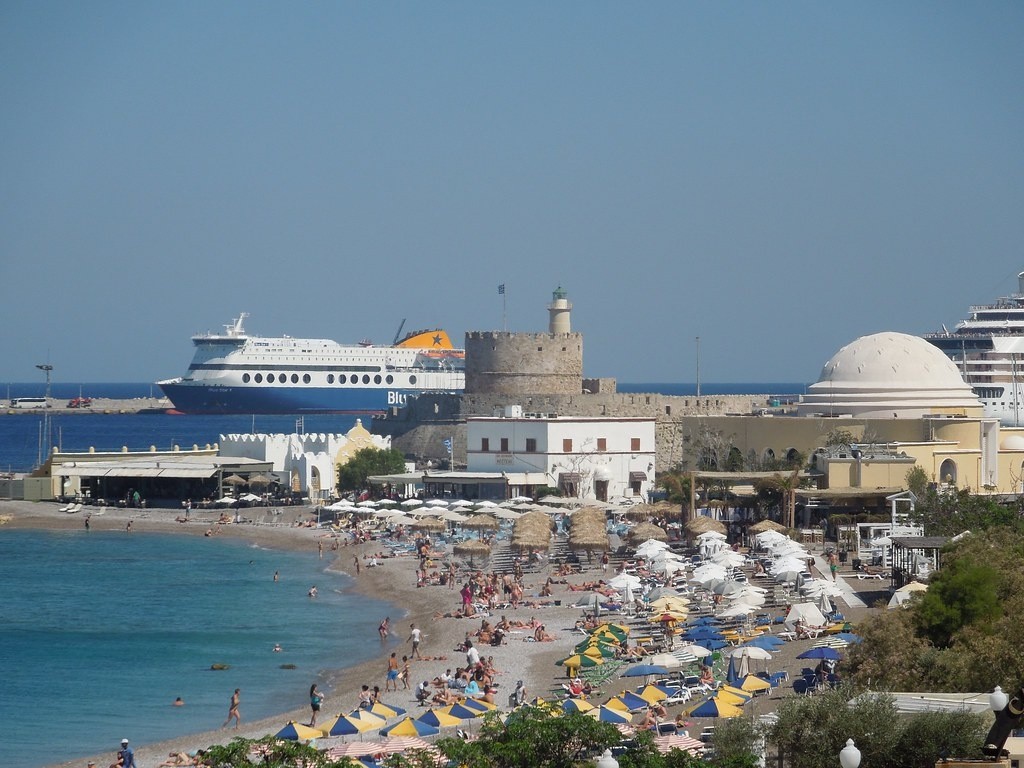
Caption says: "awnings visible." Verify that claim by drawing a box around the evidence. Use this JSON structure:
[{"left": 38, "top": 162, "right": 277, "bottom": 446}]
[{"left": 53, "top": 463, "right": 219, "bottom": 479}]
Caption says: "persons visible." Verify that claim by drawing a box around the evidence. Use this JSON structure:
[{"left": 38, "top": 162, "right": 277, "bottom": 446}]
[
  {"left": 88, "top": 738, "right": 212, "bottom": 768},
  {"left": 793, "top": 618, "right": 802, "bottom": 640},
  {"left": 173, "top": 695, "right": 184, "bottom": 706},
  {"left": 699, "top": 663, "right": 714, "bottom": 688},
  {"left": 58, "top": 489, "right": 321, "bottom": 538},
  {"left": 751, "top": 561, "right": 763, "bottom": 578},
  {"left": 786, "top": 603, "right": 792, "bottom": 616},
  {"left": 273, "top": 643, "right": 282, "bottom": 652},
  {"left": 272, "top": 571, "right": 279, "bottom": 582},
  {"left": 305, "top": 525, "right": 673, "bottom": 725},
  {"left": 641, "top": 701, "right": 667, "bottom": 728},
  {"left": 826, "top": 549, "right": 838, "bottom": 582},
  {"left": 807, "top": 550, "right": 816, "bottom": 577},
  {"left": 221, "top": 688, "right": 240, "bottom": 731}
]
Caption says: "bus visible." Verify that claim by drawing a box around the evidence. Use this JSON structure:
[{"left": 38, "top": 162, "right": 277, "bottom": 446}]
[{"left": 11, "top": 398, "right": 47, "bottom": 409}]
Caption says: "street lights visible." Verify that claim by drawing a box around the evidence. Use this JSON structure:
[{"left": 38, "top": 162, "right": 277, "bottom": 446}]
[{"left": 36, "top": 365, "right": 53, "bottom": 464}]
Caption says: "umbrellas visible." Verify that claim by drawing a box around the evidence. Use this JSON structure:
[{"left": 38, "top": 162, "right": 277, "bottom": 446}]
[
  {"left": 500, "top": 495, "right": 865, "bottom": 759},
  {"left": 319, "top": 477, "right": 533, "bottom": 582},
  {"left": 888, "top": 581, "right": 928, "bottom": 612},
  {"left": 275, "top": 698, "right": 500, "bottom": 768}
]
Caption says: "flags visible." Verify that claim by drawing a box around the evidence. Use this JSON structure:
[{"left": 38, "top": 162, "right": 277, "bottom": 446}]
[{"left": 499, "top": 284, "right": 504, "bottom": 295}]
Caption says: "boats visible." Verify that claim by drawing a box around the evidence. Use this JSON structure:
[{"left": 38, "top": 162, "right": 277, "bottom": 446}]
[
  {"left": 922, "top": 271, "right": 1024, "bottom": 429},
  {"left": 154, "top": 312, "right": 465, "bottom": 415}
]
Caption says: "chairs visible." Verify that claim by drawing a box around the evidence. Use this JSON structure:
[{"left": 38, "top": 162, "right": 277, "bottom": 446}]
[
  {"left": 319, "top": 518, "right": 549, "bottom": 574},
  {"left": 59, "top": 503, "right": 75, "bottom": 511},
  {"left": 859, "top": 566, "right": 889, "bottom": 579},
  {"left": 549, "top": 554, "right": 838, "bottom": 758},
  {"left": 95, "top": 506, "right": 106, "bottom": 515},
  {"left": 213, "top": 514, "right": 235, "bottom": 525},
  {"left": 67, "top": 504, "right": 82, "bottom": 513}
]
